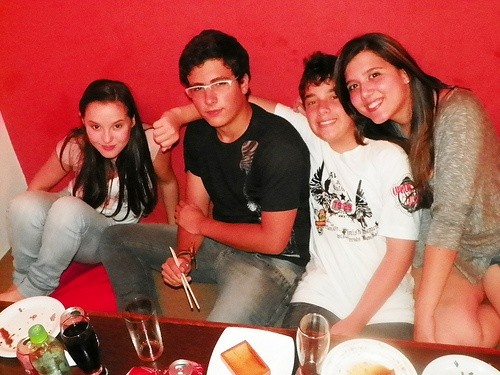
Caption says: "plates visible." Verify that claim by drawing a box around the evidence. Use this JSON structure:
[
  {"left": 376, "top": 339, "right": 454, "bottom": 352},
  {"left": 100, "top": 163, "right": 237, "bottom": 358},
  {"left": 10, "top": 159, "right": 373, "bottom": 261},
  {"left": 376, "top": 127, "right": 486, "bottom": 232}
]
[
  {"left": 421, "top": 355, "right": 500, "bottom": 375},
  {"left": 206, "top": 326, "right": 295, "bottom": 375},
  {"left": 319, "top": 339, "right": 418, "bottom": 375},
  {"left": 0, "top": 296, "right": 65, "bottom": 358}
]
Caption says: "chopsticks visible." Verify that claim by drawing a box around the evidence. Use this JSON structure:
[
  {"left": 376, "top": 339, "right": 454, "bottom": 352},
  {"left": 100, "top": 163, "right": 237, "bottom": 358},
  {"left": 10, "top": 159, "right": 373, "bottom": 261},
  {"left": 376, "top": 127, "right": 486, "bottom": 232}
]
[{"left": 169, "top": 247, "right": 200, "bottom": 309}]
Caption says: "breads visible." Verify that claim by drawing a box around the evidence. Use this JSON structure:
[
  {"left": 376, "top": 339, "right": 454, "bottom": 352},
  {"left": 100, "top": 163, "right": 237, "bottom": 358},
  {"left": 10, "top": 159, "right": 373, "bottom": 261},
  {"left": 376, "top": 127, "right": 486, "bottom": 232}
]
[{"left": 221, "top": 340, "right": 271, "bottom": 375}]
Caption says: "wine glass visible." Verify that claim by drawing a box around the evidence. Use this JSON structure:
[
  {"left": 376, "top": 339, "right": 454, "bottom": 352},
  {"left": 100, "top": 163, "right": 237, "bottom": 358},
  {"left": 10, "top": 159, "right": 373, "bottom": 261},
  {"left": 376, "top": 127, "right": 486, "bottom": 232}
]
[{"left": 296, "top": 313, "right": 330, "bottom": 375}]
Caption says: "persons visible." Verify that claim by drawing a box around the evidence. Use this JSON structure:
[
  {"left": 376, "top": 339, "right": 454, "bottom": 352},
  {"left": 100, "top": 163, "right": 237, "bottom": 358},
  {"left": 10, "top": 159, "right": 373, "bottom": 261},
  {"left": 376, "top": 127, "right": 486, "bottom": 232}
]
[
  {"left": 0, "top": 79, "right": 179, "bottom": 301},
  {"left": 152, "top": 51, "right": 420, "bottom": 337},
  {"left": 334, "top": 32, "right": 500, "bottom": 349},
  {"left": 98, "top": 29, "right": 311, "bottom": 327}
]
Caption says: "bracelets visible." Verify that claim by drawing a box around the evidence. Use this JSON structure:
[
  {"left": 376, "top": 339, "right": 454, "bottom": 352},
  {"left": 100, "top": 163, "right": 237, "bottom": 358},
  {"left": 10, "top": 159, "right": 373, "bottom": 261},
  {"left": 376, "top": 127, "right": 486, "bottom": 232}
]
[{"left": 176, "top": 249, "right": 196, "bottom": 271}]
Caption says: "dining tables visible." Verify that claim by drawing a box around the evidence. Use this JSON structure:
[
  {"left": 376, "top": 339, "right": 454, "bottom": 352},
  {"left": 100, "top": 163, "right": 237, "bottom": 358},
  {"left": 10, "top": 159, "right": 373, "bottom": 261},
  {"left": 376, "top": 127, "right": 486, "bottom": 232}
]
[{"left": 0, "top": 300, "right": 500, "bottom": 375}]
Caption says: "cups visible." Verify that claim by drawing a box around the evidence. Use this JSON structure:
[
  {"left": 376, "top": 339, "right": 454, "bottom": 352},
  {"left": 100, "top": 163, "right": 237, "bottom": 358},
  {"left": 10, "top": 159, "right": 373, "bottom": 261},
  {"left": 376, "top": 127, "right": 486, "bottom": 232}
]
[
  {"left": 122, "top": 297, "right": 163, "bottom": 361},
  {"left": 60, "top": 314, "right": 109, "bottom": 375}
]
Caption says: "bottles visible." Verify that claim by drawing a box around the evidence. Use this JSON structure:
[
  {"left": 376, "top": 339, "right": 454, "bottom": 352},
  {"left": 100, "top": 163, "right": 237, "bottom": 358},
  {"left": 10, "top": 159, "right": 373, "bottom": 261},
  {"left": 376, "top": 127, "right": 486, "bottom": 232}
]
[{"left": 28, "top": 324, "right": 71, "bottom": 375}]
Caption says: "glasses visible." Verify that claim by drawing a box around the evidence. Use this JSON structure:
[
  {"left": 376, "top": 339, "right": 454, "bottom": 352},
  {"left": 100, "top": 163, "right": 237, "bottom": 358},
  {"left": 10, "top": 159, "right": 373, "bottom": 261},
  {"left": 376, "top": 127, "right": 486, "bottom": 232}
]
[{"left": 185, "top": 76, "right": 240, "bottom": 98}]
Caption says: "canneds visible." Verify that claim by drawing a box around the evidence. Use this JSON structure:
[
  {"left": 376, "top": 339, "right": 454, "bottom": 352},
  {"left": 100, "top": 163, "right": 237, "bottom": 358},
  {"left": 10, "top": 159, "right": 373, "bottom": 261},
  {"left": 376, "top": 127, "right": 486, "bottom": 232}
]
[
  {"left": 17, "top": 337, "right": 42, "bottom": 375},
  {"left": 60, "top": 306, "right": 100, "bottom": 348}
]
